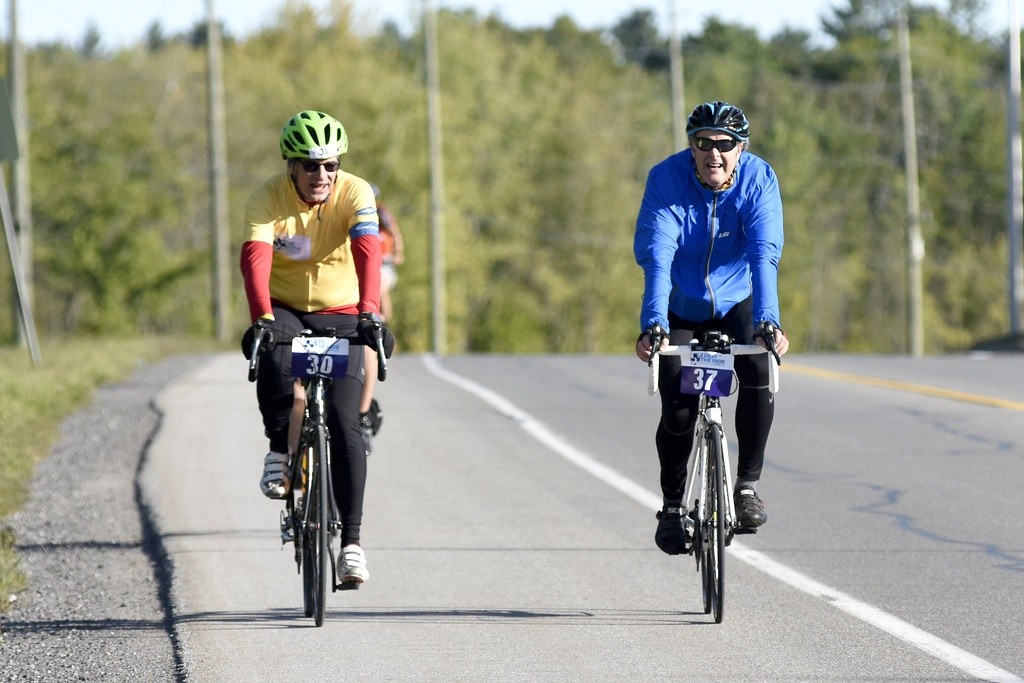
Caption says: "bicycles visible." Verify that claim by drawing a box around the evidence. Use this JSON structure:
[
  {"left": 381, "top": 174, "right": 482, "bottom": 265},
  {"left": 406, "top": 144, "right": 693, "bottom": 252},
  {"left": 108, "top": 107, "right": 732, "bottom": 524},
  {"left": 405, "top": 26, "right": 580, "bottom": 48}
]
[
  {"left": 249, "top": 311, "right": 387, "bottom": 629},
  {"left": 648, "top": 323, "right": 782, "bottom": 624}
]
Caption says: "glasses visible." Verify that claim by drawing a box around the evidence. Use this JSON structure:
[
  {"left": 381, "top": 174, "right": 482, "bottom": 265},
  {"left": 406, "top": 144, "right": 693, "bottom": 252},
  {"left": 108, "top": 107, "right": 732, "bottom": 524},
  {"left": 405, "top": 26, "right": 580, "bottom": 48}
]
[
  {"left": 297, "top": 159, "right": 341, "bottom": 172},
  {"left": 693, "top": 137, "right": 737, "bottom": 152}
]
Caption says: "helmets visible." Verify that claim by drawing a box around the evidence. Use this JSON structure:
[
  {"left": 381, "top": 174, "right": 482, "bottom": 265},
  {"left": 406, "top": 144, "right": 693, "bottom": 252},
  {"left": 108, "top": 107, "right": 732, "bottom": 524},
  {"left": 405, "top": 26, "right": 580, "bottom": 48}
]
[
  {"left": 280, "top": 109, "right": 349, "bottom": 160},
  {"left": 685, "top": 101, "right": 750, "bottom": 142}
]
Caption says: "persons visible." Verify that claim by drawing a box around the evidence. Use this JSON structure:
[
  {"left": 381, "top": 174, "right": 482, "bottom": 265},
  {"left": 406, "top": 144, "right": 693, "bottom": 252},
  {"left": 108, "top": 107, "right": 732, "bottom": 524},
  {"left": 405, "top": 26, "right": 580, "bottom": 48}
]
[
  {"left": 634, "top": 101, "right": 784, "bottom": 556},
  {"left": 239, "top": 110, "right": 382, "bottom": 585},
  {"left": 366, "top": 181, "right": 405, "bottom": 320}
]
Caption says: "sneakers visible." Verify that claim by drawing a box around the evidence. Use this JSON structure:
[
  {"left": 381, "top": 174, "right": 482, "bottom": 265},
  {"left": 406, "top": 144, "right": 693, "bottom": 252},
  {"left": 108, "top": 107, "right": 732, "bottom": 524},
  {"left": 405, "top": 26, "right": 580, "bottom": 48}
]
[
  {"left": 338, "top": 543, "right": 368, "bottom": 584},
  {"left": 260, "top": 451, "right": 290, "bottom": 498},
  {"left": 655, "top": 504, "right": 686, "bottom": 556},
  {"left": 731, "top": 485, "right": 767, "bottom": 528}
]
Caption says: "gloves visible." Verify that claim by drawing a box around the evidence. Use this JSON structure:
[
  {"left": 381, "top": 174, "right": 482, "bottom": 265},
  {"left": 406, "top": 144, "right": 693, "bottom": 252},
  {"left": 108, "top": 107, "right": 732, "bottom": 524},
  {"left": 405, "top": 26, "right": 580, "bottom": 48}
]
[
  {"left": 241, "top": 318, "right": 277, "bottom": 360},
  {"left": 357, "top": 319, "right": 394, "bottom": 358}
]
